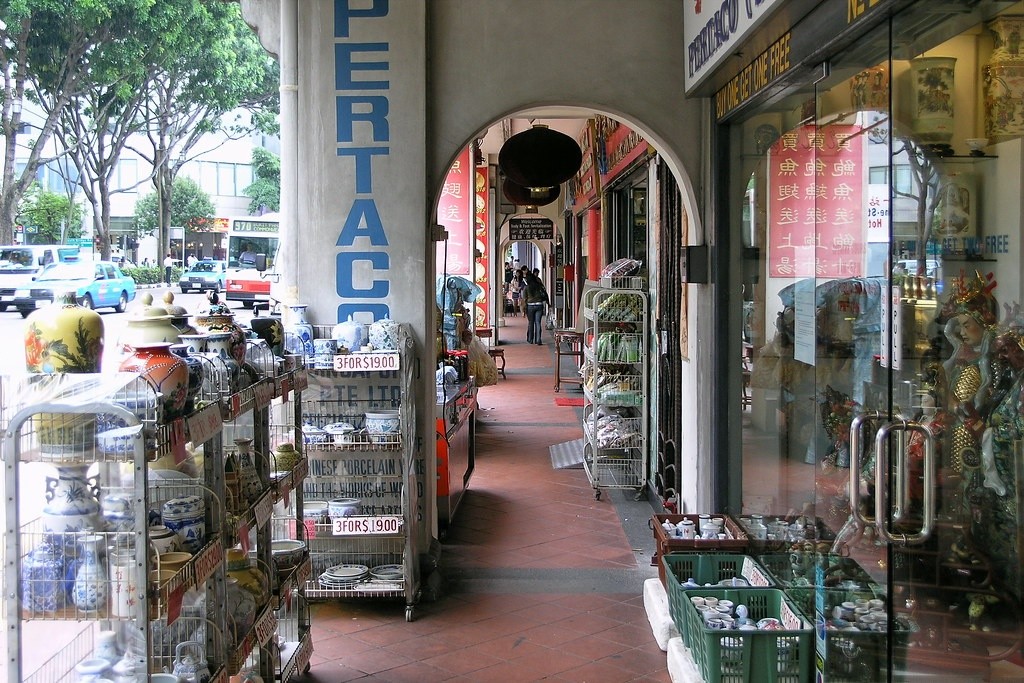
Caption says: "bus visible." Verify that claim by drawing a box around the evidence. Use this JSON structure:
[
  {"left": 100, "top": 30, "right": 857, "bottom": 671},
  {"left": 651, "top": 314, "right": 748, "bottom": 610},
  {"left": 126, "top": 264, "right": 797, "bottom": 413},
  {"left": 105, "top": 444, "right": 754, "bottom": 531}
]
[{"left": 224, "top": 211, "right": 282, "bottom": 312}]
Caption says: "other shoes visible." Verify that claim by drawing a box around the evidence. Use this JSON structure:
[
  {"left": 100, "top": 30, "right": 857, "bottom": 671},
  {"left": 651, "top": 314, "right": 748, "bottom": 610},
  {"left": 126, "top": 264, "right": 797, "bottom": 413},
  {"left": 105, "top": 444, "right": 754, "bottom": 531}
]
[
  {"left": 536, "top": 343, "right": 543, "bottom": 346},
  {"left": 522, "top": 312, "right": 525, "bottom": 318},
  {"left": 529, "top": 340, "right": 533, "bottom": 344}
]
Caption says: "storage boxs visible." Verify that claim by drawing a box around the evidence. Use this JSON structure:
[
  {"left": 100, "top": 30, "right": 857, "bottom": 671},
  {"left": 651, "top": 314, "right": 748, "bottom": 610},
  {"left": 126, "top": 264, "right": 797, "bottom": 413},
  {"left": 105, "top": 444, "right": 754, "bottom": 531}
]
[
  {"left": 756, "top": 555, "right": 879, "bottom": 588},
  {"left": 683, "top": 589, "right": 815, "bottom": 683},
  {"left": 733, "top": 515, "right": 847, "bottom": 555},
  {"left": 785, "top": 589, "right": 911, "bottom": 683},
  {"left": 662, "top": 556, "right": 776, "bottom": 633},
  {"left": 648, "top": 513, "right": 747, "bottom": 590},
  {"left": 598, "top": 276, "right": 643, "bottom": 289}
]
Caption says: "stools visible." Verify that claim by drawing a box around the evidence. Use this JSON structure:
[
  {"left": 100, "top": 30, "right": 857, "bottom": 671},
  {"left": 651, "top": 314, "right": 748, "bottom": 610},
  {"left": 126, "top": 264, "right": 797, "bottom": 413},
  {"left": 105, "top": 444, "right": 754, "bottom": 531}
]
[
  {"left": 488, "top": 348, "right": 507, "bottom": 380},
  {"left": 476, "top": 328, "right": 492, "bottom": 349}
]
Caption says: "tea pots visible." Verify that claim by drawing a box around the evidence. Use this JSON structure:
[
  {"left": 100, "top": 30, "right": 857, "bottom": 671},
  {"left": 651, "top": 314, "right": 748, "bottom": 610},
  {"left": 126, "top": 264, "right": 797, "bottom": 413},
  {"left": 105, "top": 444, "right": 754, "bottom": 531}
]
[{"left": 162, "top": 641, "right": 211, "bottom": 683}]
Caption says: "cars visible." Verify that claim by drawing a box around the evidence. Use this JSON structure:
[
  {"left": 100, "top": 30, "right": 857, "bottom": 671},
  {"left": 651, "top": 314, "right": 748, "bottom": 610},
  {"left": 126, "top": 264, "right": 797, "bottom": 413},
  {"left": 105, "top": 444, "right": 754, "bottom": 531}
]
[
  {"left": 11, "top": 255, "right": 138, "bottom": 317},
  {"left": 112, "top": 255, "right": 138, "bottom": 272},
  {"left": 178, "top": 256, "right": 226, "bottom": 294}
]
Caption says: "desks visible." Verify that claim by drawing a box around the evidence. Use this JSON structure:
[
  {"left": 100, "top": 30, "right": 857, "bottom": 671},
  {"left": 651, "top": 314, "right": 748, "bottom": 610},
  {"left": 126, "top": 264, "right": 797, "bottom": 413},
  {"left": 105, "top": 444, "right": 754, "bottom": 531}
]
[{"left": 554, "top": 328, "right": 582, "bottom": 392}]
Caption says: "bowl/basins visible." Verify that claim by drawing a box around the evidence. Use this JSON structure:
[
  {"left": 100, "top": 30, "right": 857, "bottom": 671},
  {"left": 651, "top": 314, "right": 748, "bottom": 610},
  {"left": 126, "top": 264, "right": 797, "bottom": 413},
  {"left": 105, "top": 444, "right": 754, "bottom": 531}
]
[
  {"left": 255, "top": 539, "right": 306, "bottom": 585},
  {"left": 291, "top": 498, "right": 362, "bottom": 522}
]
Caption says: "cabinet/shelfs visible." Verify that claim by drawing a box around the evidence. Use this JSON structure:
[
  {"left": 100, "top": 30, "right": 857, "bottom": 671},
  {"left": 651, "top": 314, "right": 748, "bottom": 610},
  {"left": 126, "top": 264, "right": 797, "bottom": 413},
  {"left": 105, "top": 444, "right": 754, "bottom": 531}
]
[
  {"left": 434, "top": 374, "right": 478, "bottom": 527},
  {"left": 6, "top": 324, "right": 421, "bottom": 683},
  {"left": 584, "top": 288, "right": 652, "bottom": 501}
]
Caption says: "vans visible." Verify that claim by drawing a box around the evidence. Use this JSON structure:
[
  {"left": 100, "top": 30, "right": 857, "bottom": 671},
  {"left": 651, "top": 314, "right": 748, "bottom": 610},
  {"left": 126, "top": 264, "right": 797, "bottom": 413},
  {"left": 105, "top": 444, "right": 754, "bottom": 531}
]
[{"left": 0, "top": 244, "right": 81, "bottom": 312}]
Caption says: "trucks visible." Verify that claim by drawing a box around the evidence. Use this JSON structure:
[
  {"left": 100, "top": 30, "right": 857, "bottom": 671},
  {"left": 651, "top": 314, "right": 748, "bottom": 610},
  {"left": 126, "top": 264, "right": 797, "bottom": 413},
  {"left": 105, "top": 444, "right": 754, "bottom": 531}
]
[{"left": 255, "top": 237, "right": 282, "bottom": 323}]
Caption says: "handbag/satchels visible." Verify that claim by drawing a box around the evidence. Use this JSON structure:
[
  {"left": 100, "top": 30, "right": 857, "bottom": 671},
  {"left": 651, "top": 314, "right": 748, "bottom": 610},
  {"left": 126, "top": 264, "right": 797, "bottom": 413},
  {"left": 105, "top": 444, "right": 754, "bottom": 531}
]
[
  {"left": 512, "top": 292, "right": 519, "bottom": 298},
  {"left": 538, "top": 283, "right": 548, "bottom": 301},
  {"left": 468, "top": 334, "right": 498, "bottom": 387}
]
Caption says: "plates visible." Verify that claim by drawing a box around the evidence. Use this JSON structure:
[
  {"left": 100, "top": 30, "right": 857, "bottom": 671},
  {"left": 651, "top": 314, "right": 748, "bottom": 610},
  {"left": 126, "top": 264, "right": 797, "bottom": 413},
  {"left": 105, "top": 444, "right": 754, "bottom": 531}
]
[{"left": 318, "top": 563, "right": 404, "bottom": 590}]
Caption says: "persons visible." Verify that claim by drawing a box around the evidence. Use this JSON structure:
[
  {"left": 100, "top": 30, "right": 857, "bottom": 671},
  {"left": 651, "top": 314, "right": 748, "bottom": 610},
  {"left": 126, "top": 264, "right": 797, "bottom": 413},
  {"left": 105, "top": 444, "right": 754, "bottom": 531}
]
[
  {"left": 145, "top": 258, "right": 156, "bottom": 269},
  {"left": 187, "top": 253, "right": 199, "bottom": 270},
  {"left": 989, "top": 328, "right": 1024, "bottom": 514},
  {"left": 504, "top": 256, "right": 539, "bottom": 317},
  {"left": 239, "top": 243, "right": 257, "bottom": 265},
  {"left": 164, "top": 253, "right": 182, "bottom": 287},
  {"left": 903, "top": 385, "right": 952, "bottom": 463},
  {"left": 949, "top": 268, "right": 1003, "bottom": 473},
  {"left": 523, "top": 271, "right": 549, "bottom": 345}
]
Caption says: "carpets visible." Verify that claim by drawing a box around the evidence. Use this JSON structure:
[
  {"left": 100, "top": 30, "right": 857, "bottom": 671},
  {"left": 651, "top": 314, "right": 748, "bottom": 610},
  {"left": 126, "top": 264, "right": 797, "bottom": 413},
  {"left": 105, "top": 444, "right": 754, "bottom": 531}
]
[{"left": 555, "top": 397, "right": 591, "bottom": 406}]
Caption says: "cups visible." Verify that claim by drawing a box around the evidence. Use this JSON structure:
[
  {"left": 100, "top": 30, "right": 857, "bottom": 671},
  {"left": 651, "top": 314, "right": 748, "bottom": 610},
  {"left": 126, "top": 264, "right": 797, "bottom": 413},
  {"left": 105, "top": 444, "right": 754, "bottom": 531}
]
[
  {"left": 691, "top": 595, "right": 779, "bottom": 631},
  {"left": 825, "top": 598, "right": 887, "bottom": 630},
  {"left": 313, "top": 340, "right": 336, "bottom": 369},
  {"left": 108, "top": 548, "right": 139, "bottom": 617},
  {"left": 741, "top": 513, "right": 805, "bottom": 541},
  {"left": 662, "top": 514, "right": 726, "bottom": 541},
  {"left": 147, "top": 552, "right": 192, "bottom": 612}
]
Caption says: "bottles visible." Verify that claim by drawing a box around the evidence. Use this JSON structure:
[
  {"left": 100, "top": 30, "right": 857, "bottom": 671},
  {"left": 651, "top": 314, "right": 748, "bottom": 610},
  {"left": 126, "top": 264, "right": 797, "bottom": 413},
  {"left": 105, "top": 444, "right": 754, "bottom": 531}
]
[{"left": 72, "top": 536, "right": 108, "bottom": 612}]
